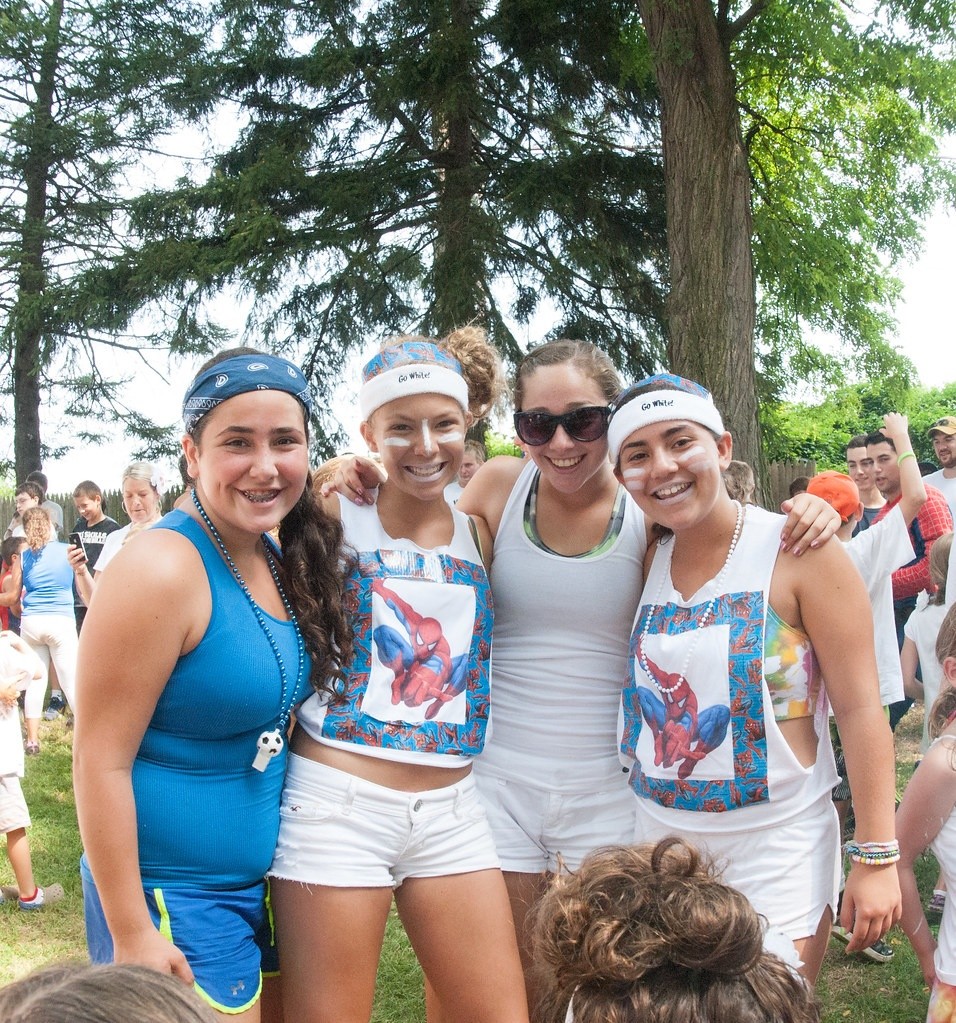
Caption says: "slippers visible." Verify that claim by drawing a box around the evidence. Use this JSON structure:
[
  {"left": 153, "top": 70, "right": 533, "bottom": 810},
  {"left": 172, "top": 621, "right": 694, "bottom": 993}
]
[
  {"left": 0, "top": 884, "right": 19, "bottom": 903},
  {"left": 17, "top": 882, "right": 65, "bottom": 913}
]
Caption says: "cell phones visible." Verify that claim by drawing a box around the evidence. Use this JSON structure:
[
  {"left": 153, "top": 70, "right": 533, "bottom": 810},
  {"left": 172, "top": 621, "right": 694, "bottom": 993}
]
[{"left": 69, "top": 532, "right": 87, "bottom": 562}]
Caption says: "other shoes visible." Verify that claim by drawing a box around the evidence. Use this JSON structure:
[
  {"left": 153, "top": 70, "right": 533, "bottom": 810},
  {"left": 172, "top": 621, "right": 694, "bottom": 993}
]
[
  {"left": 44, "top": 697, "right": 65, "bottom": 721},
  {"left": 26, "top": 730, "right": 39, "bottom": 754},
  {"left": 831, "top": 915, "right": 896, "bottom": 961},
  {"left": 925, "top": 894, "right": 945, "bottom": 914},
  {"left": 842, "top": 791, "right": 902, "bottom": 839}
]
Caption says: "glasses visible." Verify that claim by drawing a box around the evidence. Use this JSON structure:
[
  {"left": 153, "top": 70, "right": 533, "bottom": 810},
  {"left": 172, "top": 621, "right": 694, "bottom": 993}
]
[
  {"left": 928, "top": 419, "right": 956, "bottom": 430},
  {"left": 513, "top": 402, "right": 613, "bottom": 446}
]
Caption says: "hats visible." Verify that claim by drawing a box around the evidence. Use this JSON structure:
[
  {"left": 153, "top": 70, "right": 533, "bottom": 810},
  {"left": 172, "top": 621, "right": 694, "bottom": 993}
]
[
  {"left": 928, "top": 416, "right": 956, "bottom": 438},
  {"left": 807, "top": 470, "right": 860, "bottom": 522}
]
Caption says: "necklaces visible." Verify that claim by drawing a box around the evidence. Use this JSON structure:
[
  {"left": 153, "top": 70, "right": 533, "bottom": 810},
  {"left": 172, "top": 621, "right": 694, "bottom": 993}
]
[
  {"left": 188, "top": 484, "right": 305, "bottom": 772},
  {"left": 638, "top": 500, "right": 742, "bottom": 693}
]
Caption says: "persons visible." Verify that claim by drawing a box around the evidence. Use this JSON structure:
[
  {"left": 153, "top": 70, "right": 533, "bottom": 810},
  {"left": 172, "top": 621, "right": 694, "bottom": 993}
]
[
  {"left": 790, "top": 415, "right": 956, "bottom": 728},
  {"left": 1, "top": 420, "right": 488, "bottom": 1023},
  {"left": 322, "top": 338, "right": 842, "bottom": 1023},
  {"left": 0, "top": 958, "right": 226, "bottom": 1023},
  {"left": 605, "top": 373, "right": 902, "bottom": 1008},
  {"left": 268, "top": 328, "right": 532, "bottom": 1023},
  {"left": 73, "top": 346, "right": 313, "bottom": 1023},
  {"left": 909, "top": 535, "right": 954, "bottom": 913},
  {"left": 895, "top": 602, "right": 956, "bottom": 1023},
  {"left": 524, "top": 840, "right": 823, "bottom": 1023},
  {"left": 805, "top": 413, "right": 928, "bottom": 964}
]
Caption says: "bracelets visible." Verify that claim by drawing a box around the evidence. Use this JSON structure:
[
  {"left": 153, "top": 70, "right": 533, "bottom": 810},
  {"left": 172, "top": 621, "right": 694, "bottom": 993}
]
[
  {"left": 845, "top": 840, "right": 900, "bottom": 865},
  {"left": 898, "top": 452, "right": 916, "bottom": 467}
]
